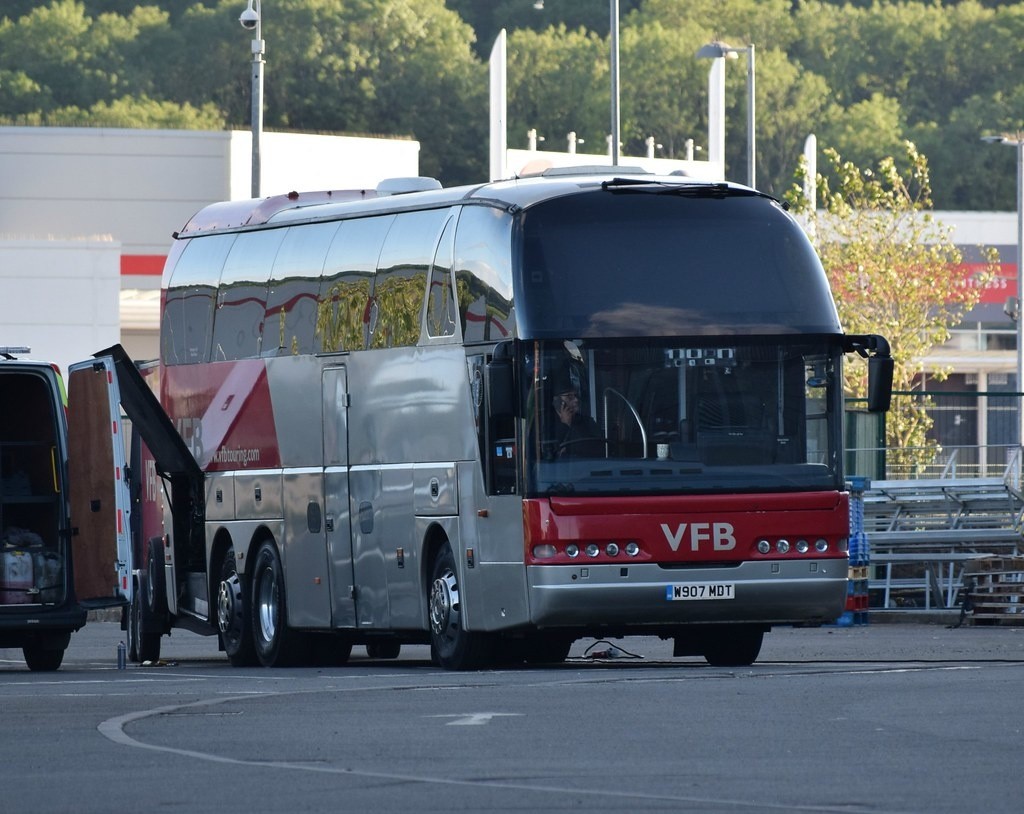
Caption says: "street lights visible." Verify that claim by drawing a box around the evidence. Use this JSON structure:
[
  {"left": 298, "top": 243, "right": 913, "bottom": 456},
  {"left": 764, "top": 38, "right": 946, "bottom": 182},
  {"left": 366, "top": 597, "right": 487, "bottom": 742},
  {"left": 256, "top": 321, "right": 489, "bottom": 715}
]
[
  {"left": 993, "top": 137, "right": 1022, "bottom": 443},
  {"left": 238, "top": 0, "right": 267, "bottom": 200},
  {"left": 695, "top": 42, "right": 757, "bottom": 191}
]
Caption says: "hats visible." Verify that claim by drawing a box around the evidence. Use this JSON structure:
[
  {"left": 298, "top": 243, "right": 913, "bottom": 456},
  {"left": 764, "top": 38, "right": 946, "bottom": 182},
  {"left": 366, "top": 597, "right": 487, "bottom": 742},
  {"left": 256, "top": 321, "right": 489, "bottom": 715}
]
[{"left": 549, "top": 371, "right": 583, "bottom": 396}]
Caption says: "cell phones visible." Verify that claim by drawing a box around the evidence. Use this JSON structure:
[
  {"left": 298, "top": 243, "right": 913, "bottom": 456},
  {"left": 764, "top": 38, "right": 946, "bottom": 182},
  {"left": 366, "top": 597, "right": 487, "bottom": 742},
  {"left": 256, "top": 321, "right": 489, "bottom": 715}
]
[{"left": 553, "top": 398, "right": 567, "bottom": 412}]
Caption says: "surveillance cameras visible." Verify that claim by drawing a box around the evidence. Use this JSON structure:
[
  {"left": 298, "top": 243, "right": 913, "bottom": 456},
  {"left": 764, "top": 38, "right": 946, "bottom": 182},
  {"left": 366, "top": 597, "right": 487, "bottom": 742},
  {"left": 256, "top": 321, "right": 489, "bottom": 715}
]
[{"left": 239, "top": 9, "right": 260, "bottom": 29}]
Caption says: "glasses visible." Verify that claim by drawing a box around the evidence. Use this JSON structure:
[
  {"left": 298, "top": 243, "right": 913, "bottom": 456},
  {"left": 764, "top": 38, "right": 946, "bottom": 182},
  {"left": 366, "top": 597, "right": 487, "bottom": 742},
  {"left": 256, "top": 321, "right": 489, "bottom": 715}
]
[{"left": 560, "top": 394, "right": 581, "bottom": 399}]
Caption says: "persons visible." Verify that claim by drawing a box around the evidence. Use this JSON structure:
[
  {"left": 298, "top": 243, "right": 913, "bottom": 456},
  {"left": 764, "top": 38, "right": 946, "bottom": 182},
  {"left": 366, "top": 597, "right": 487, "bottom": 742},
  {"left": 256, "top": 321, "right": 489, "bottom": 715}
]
[{"left": 529, "top": 379, "right": 604, "bottom": 459}]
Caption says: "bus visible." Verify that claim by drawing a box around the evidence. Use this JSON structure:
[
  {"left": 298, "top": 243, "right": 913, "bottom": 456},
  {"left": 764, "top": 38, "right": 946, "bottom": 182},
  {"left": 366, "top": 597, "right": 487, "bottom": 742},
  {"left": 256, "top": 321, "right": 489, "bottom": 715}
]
[
  {"left": 122, "top": 359, "right": 168, "bottom": 664},
  {"left": 158, "top": 165, "right": 893, "bottom": 674}
]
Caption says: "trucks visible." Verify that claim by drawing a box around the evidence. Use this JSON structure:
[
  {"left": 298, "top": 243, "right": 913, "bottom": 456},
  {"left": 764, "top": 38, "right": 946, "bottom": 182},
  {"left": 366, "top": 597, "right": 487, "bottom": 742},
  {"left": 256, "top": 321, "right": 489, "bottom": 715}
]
[{"left": 0, "top": 342, "right": 130, "bottom": 673}]
